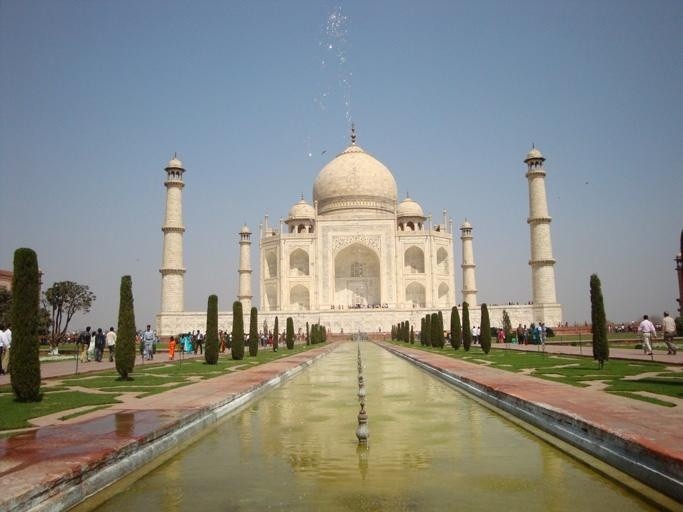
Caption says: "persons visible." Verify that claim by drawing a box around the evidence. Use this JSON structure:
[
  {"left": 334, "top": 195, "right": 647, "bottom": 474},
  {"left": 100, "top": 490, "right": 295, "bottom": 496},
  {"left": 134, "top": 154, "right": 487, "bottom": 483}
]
[
  {"left": 330, "top": 303, "right": 389, "bottom": 310},
  {"left": 412, "top": 321, "right": 547, "bottom": 348},
  {"left": 607, "top": 320, "right": 661, "bottom": 335},
  {"left": 38, "top": 333, "right": 75, "bottom": 347},
  {"left": 660, "top": 311, "right": 677, "bottom": 355},
  {"left": 1, "top": 323, "right": 12, "bottom": 375},
  {"left": 0, "top": 324, "right": 11, "bottom": 375},
  {"left": 76, "top": 323, "right": 306, "bottom": 363},
  {"left": 636, "top": 314, "right": 657, "bottom": 355}
]
